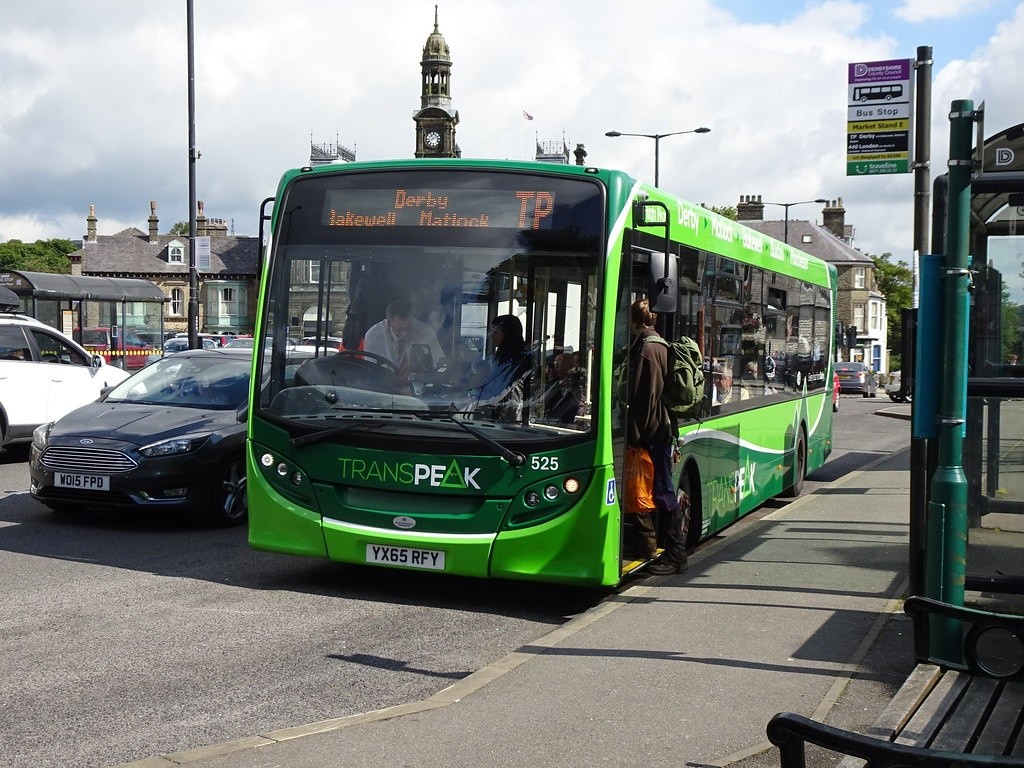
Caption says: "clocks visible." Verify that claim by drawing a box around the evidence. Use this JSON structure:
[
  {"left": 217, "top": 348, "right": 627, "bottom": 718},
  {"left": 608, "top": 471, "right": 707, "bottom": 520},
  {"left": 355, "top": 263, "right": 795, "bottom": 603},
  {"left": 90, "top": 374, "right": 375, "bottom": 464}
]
[{"left": 424, "top": 131, "right": 442, "bottom": 148}]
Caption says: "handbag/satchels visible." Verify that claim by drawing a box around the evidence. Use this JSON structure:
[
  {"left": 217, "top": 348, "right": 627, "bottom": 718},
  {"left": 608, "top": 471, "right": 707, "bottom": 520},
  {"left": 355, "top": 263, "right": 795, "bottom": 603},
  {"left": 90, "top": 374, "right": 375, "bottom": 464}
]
[{"left": 622, "top": 447, "right": 656, "bottom": 514}]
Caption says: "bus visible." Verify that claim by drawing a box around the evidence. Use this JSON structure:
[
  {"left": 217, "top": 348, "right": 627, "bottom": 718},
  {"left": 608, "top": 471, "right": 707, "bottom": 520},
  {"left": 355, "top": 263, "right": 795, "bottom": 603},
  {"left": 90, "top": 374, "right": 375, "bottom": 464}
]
[
  {"left": 244, "top": 158, "right": 837, "bottom": 591},
  {"left": 704, "top": 324, "right": 742, "bottom": 357}
]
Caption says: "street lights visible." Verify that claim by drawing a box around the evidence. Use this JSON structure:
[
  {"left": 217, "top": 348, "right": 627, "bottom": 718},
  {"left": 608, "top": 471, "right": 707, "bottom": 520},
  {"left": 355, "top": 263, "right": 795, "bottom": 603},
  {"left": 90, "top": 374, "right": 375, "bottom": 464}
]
[
  {"left": 748, "top": 198, "right": 828, "bottom": 245},
  {"left": 605, "top": 128, "right": 711, "bottom": 189}
]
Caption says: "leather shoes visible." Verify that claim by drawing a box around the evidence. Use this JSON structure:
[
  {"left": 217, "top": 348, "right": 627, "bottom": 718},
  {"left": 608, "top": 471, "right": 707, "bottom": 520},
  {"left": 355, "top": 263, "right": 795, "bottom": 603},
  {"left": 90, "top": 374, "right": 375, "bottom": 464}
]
[
  {"left": 622, "top": 545, "right": 657, "bottom": 560},
  {"left": 648, "top": 560, "right": 688, "bottom": 574}
]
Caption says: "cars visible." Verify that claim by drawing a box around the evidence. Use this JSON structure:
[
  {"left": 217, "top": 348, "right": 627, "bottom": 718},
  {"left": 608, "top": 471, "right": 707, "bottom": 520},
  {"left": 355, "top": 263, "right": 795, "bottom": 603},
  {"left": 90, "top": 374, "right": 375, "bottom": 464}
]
[
  {"left": 835, "top": 362, "right": 877, "bottom": 397},
  {"left": 145, "top": 336, "right": 216, "bottom": 370},
  {"left": 885, "top": 369, "right": 912, "bottom": 403},
  {"left": 29, "top": 346, "right": 307, "bottom": 527},
  {"left": 831, "top": 372, "right": 841, "bottom": 411},
  {"left": 295, "top": 335, "right": 342, "bottom": 355},
  {"left": 224, "top": 338, "right": 296, "bottom": 347}
]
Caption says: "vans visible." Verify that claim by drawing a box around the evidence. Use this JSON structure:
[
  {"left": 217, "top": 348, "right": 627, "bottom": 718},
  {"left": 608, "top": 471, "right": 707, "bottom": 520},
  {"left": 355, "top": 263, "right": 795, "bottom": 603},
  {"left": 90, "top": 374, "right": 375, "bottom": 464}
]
[{"left": 171, "top": 331, "right": 251, "bottom": 349}]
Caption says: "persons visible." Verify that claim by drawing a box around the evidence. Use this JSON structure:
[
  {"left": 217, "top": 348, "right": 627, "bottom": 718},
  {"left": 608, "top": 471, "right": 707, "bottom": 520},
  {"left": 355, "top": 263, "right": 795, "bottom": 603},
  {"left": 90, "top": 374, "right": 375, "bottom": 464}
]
[
  {"left": 476, "top": 315, "right": 527, "bottom": 401},
  {"left": 711, "top": 360, "right": 774, "bottom": 407},
  {"left": 1009, "top": 355, "right": 1017, "bottom": 365},
  {"left": 364, "top": 299, "right": 449, "bottom": 397},
  {"left": 435, "top": 349, "right": 581, "bottom": 425},
  {"left": 630, "top": 299, "right": 689, "bottom": 575}
]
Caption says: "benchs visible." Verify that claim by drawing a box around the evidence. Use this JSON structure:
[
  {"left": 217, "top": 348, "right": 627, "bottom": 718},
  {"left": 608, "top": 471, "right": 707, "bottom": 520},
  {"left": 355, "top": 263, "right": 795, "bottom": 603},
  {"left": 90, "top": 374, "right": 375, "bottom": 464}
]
[{"left": 766, "top": 594, "right": 1024, "bottom": 768}]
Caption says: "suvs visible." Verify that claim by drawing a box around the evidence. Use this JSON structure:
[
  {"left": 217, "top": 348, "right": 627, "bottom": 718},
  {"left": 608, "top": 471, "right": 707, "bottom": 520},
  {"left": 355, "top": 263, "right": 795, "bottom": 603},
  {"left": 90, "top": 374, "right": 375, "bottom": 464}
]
[
  {"left": 0, "top": 286, "right": 147, "bottom": 453},
  {"left": 73, "top": 325, "right": 156, "bottom": 369}
]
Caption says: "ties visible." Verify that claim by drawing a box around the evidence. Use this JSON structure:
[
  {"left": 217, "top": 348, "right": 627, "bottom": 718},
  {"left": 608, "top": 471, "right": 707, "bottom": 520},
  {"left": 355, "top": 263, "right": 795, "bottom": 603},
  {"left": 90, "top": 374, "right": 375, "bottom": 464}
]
[{"left": 397, "top": 339, "right": 410, "bottom": 386}]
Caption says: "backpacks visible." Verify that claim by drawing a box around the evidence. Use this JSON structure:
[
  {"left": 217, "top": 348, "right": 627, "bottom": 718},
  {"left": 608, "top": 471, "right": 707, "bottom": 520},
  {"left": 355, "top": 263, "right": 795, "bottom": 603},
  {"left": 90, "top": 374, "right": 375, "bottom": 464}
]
[{"left": 639, "top": 336, "right": 705, "bottom": 419}]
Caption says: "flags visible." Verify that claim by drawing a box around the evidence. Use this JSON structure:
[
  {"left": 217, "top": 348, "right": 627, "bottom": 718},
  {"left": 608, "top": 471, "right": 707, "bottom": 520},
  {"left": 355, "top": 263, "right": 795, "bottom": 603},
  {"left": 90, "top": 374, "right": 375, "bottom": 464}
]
[{"left": 522, "top": 110, "right": 533, "bottom": 120}]
